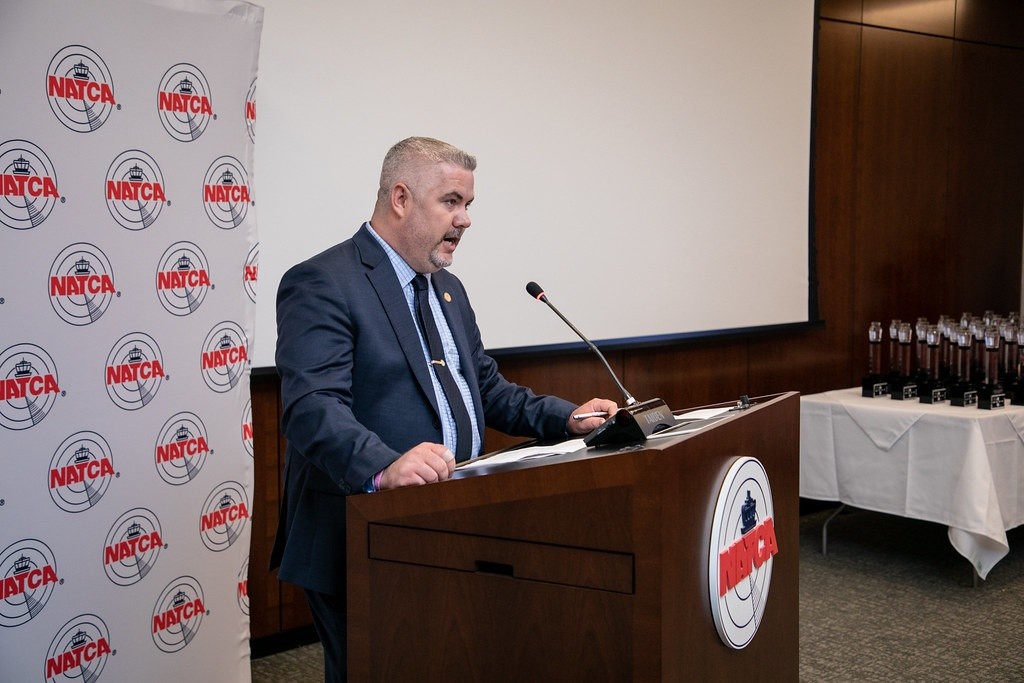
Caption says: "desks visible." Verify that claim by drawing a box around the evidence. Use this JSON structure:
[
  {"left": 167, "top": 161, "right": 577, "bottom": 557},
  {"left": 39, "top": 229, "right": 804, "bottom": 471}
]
[{"left": 801, "top": 388, "right": 1024, "bottom": 588}]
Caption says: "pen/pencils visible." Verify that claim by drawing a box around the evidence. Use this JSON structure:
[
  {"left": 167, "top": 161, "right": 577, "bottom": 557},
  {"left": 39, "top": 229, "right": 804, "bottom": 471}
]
[{"left": 573, "top": 410, "right": 608, "bottom": 420}]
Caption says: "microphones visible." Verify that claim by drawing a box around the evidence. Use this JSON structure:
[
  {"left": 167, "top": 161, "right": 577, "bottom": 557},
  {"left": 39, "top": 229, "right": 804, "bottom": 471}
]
[{"left": 526, "top": 282, "right": 676, "bottom": 448}]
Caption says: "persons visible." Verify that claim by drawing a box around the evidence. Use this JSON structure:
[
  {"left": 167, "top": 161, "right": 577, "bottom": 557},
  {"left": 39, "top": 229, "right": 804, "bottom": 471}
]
[{"left": 268, "top": 136, "right": 618, "bottom": 683}]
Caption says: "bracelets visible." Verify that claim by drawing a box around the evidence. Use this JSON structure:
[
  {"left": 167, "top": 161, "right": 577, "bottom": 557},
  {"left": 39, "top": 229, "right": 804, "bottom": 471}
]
[{"left": 373, "top": 470, "right": 384, "bottom": 490}]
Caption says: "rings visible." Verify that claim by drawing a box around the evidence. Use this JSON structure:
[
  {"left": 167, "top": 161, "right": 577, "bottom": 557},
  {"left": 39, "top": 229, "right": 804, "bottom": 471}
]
[{"left": 441, "top": 449, "right": 454, "bottom": 461}]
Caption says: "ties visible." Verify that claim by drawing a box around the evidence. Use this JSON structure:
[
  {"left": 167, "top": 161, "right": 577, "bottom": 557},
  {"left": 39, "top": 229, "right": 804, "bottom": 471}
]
[{"left": 411, "top": 274, "right": 472, "bottom": 464}]
